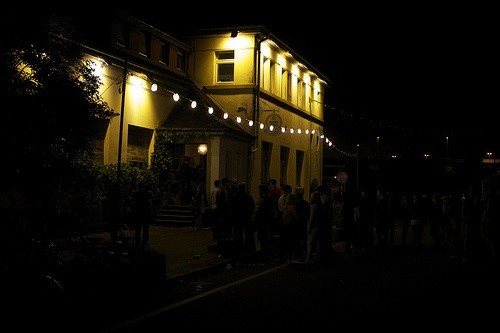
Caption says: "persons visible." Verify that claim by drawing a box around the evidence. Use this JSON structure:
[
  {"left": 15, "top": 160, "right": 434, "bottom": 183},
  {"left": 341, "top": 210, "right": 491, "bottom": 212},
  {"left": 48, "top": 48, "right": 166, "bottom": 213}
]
[
  {"left": 191, "top": 182, "right": 206, "bottom": 230},
  {"left": 229, "top": 181, "right": 240, "bottom": 197},
  {"left": 208, "top": 179, "right": 226, "bottom": 251},
  {"left": 257, "top": 175, "right": 500, "bottom": 262},
  {"left": 221, "top": 177, "right": 233, "bottom": 244},
  {"left": 106, "top": 183, "right": 121, "bottom": 241},
  {"left": 132, "top": 182, "right": 149, "bottom": 248},
  {"left": 232, "top": 183, "right": 255, "bottom": 251}
]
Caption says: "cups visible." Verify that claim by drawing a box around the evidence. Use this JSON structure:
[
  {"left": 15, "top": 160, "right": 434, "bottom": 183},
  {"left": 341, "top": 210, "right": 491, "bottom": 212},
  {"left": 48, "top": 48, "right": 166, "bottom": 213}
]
[{"left": 192, "top": 254, "right": 200, "bottom": 259}]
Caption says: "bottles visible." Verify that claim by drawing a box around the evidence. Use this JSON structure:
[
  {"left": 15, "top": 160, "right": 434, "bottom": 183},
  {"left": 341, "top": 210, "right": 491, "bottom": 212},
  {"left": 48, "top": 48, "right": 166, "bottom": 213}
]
[{"left": 216, "top": 254, "right": 222, "bottom": 261}]
